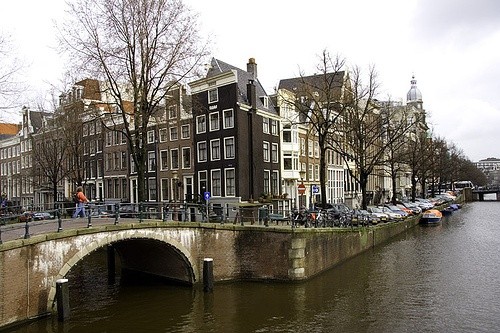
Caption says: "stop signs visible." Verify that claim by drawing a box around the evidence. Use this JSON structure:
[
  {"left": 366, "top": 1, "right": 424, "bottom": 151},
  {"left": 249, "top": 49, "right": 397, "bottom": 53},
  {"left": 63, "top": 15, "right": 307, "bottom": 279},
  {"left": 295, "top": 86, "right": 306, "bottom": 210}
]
[{"left": 297, "top": 184, "right": 306, "bottom": 194}]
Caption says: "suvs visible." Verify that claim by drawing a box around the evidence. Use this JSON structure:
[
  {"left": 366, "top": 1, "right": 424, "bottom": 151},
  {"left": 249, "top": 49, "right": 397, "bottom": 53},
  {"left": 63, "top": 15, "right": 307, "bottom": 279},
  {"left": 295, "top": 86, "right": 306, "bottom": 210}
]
[{"left": 313, "top": 201, "right": 350, "bottom": 226}]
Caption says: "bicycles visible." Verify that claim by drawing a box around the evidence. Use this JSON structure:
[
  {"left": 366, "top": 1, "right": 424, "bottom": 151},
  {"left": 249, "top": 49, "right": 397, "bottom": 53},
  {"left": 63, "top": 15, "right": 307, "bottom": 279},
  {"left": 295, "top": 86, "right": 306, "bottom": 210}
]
[{"left": 288, "top": 207, "right": 354, "bottom": 228}]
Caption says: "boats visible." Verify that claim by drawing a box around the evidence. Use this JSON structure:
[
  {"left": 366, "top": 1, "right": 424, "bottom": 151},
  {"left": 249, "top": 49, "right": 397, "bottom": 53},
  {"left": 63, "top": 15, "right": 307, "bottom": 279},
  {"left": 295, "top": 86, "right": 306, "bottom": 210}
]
[
  {"left": 450, "top": 204, "right": 459, "bottom": 211},
  {"left": 421, "top": 208, "right": 443, "bottom": 224},
  {"left": 443, "top": 207, "right": 454, "bottom": 215},
  {"left": 456, "top": 203, "right": 463, "bottom": 209}
]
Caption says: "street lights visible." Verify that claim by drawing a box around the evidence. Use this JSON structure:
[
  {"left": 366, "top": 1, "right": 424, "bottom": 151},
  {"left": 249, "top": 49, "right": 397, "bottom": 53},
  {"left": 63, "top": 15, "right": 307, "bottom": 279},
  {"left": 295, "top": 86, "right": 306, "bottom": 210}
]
[
  {"left": 298, "top": 170, "right": 307, "bottom": 207},
  {"left": 171, "top": 170, "right": 179, "bottom": 221},
  {"left": 375, "top": 185, "right": 380, "bottom": 207}
]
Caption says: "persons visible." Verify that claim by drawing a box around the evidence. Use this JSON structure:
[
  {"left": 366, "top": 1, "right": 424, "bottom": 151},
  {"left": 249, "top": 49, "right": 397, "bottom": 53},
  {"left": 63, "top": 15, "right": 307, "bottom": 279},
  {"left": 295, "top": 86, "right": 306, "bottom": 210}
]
[
  {"left": 309, "top": 213, "right": 315, "bottom": 228},
  {"left": 71, "top": 187, "right": 89, "bottom": 219},
  {"left": 291, "top": 211, "right": 304, "bottom": 228}
]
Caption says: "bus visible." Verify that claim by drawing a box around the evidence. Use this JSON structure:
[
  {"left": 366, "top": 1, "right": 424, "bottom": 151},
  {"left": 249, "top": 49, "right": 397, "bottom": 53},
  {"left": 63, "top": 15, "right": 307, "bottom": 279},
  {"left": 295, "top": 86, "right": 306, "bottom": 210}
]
[{"left": 438, "top": 181, "right": 475, "bottom": 194}]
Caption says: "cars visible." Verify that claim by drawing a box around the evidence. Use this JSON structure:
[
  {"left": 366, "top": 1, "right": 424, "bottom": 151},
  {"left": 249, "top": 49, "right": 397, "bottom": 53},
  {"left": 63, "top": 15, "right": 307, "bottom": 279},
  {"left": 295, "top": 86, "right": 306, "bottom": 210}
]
[
  {"left": 18, "top": 210, "right": 52, "bottom": 223},
  {"left": 351, "top": 190, "right": 457, "bottom": 226},
  {"left": 86, "top": 208, "right": 139, "bottom": 218}
]
